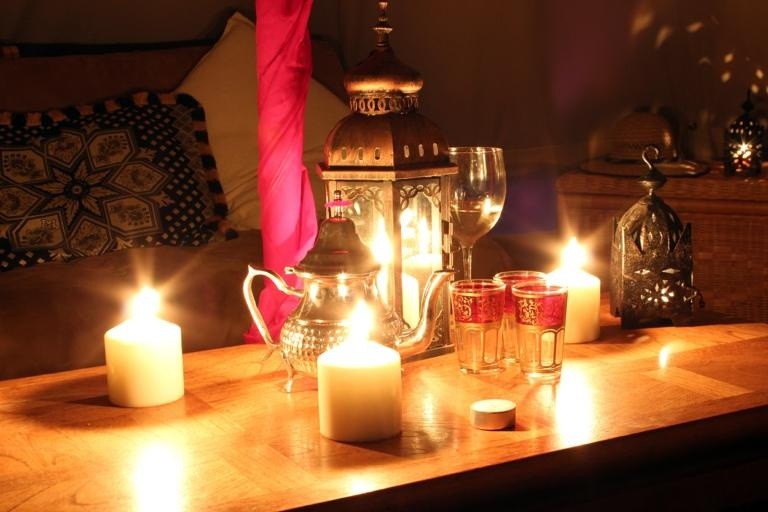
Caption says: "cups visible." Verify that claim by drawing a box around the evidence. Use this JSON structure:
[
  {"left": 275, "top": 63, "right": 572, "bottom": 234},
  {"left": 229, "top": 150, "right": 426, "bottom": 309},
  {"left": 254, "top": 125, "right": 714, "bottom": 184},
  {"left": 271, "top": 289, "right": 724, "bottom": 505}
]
[{"left": 448, "top": 270, "right": 567, "bottom": 385}]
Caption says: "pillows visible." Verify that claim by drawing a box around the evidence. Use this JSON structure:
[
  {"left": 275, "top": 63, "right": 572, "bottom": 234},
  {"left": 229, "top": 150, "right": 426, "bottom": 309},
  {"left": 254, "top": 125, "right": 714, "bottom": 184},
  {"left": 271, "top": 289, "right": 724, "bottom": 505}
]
[
  {"left": 174, "top": 13, "right": 351, "bottom": 228},
  {"left": 0, "top": 91, "right": 235, "bottom": 268}
]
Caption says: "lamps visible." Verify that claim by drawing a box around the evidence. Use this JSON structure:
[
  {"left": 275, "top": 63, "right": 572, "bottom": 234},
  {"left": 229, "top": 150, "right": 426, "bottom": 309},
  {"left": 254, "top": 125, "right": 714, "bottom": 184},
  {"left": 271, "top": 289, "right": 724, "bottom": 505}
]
[
  {"left": 315, "top": 0, "right": 460, "bottom": 359},
  {"left": 724, "top": 89, "right": 768, "bottom": 175}
]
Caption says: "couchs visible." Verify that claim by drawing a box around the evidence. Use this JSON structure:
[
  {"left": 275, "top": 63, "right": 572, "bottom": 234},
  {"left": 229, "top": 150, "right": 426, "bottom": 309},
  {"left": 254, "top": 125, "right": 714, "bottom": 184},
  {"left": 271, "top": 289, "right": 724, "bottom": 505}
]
[{"left": 0, "top": 44, "right": 337, "bottom": 375}]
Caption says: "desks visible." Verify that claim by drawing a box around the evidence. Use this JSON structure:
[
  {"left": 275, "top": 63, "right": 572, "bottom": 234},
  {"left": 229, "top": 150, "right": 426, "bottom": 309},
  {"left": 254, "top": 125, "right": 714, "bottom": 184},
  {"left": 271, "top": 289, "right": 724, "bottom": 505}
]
[{"left": 0, "top": 293, "right": 768, "bottom": 511}]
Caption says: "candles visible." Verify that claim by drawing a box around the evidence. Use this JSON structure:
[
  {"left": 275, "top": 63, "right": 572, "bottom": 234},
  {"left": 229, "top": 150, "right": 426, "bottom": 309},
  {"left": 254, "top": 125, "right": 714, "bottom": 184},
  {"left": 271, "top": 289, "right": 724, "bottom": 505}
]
[
  {"left": 106, "top": 278, "right": 184, "bottom": 405},
  {"left": 314, "top": 307, "right": 400, "bottom": 442},
  {"left": 548, "top": 242, "right": 600, "bottom": 342}
]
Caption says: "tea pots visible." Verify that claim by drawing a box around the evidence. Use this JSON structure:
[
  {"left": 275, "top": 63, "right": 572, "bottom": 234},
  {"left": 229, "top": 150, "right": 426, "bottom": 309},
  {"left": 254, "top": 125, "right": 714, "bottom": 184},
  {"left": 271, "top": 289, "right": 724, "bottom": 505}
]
[{"left": 244, "top": 190, "right": 458, "bottom": 394}]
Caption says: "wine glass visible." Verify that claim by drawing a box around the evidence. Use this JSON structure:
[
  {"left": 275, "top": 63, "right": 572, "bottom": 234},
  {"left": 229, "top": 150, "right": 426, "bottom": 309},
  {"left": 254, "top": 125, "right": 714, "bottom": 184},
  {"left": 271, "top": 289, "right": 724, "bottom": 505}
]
[{"left": 448, "top": 147, "right": 505, "bottom": 281}]
